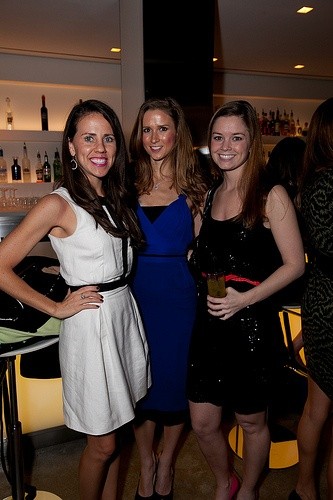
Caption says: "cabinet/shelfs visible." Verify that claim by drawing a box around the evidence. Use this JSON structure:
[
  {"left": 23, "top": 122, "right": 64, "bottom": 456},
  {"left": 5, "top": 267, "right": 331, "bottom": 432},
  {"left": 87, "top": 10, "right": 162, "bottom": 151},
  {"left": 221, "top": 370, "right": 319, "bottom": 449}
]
[
  {"left": 212, "top": 94, "right": 326, "bottom": 146},
  {"left": 0, "top": 79, "right": 122, "bottom": 212}
]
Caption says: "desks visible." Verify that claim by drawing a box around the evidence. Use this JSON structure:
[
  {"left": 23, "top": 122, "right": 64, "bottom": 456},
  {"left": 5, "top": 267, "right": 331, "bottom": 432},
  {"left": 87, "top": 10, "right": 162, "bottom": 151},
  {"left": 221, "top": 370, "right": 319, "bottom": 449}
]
[{"left": 1, "top": 337, "right": 71, "bottom": 500}]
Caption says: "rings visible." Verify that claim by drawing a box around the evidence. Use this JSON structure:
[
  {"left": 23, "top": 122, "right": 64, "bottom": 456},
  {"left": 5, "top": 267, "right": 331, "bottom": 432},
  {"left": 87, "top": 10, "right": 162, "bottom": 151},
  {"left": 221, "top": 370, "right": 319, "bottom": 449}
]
[{"left": 81, "top": 294, "right": 84, "bottom": 299}]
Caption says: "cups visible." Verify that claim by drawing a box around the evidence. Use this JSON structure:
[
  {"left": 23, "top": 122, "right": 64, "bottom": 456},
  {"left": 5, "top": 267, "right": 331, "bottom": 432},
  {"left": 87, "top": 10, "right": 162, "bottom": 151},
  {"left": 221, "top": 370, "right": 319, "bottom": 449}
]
[
  {"left": 16, "top": 196, "right": 42, "bottom": 209},
  {"left": 207, "top": 272, "right": 227, "bottom": 318}
]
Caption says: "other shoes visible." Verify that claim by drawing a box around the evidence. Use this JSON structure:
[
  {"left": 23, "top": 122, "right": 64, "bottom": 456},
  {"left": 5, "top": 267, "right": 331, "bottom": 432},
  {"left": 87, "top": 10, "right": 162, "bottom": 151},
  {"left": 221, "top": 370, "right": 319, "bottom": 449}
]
[
  {"left": 229, "top": 471, "right": 240, "bottom": 500},
  {"left": 134, "top": 473, "right": 156, "bottom": 500},
  {"left": 154, "top": 475, "right": 174, "bottom": 500}
]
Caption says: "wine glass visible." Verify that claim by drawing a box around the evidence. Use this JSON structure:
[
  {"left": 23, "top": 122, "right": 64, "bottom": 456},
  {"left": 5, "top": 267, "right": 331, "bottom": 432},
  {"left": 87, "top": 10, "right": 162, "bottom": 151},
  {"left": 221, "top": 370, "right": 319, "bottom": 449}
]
[{"left": 0, "top": 186, "right": 19, "bottom": 207}]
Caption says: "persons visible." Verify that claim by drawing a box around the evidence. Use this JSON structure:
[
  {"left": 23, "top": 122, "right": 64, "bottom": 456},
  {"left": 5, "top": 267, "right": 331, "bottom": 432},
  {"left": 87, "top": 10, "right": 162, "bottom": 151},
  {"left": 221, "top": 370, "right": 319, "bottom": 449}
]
[
  {"left": 0, "top": 100, "right": 154, "bottom": 500},
  {"left": 271, "top": 137, "right": 306, "bottom": 368},
  {"left": 289, "top": 96, "right": 333, "bottom": 500},
  {"left": 186, "top": 99, "right": 305, "bottom": 500},
  {"left": 129, "top": 98, "right": 205, "bottom": 500}
]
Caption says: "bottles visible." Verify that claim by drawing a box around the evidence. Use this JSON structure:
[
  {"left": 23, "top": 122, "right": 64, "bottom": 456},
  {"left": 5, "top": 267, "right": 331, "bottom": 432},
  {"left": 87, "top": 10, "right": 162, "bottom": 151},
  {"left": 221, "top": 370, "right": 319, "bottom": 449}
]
[
  {"left": 252, "top": 106, "right": 309, "bottom": 140},
  {"left": 23, "top": 143, "right": 31, "bottom": 183},
  {"left": 40, "top": 95, "right": 49, "bottom": 131},
  {"left": 43, "top": 151, "right": 51, "bottom": 183},
  {"left": 10, "top": 155, "right": 22, "bottom": 184},
  {"left": 35, "top": 150, "right": 43, "bottom": 183},
  {"left": 5, "top": 97, "right": 14, "bottom": 130},
  {"left": 6, "top": 149, "right": 8, "bottom": 180},
  {"left": 53, "top": 148, "right": 62, "bottom": 182}
]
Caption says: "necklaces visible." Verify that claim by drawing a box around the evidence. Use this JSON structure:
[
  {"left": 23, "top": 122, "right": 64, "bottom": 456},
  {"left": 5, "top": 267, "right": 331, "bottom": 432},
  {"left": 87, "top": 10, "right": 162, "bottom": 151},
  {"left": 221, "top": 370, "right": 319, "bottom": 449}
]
[{"left": 154, "top": 182, "right": 163, "bottom": 189}]
